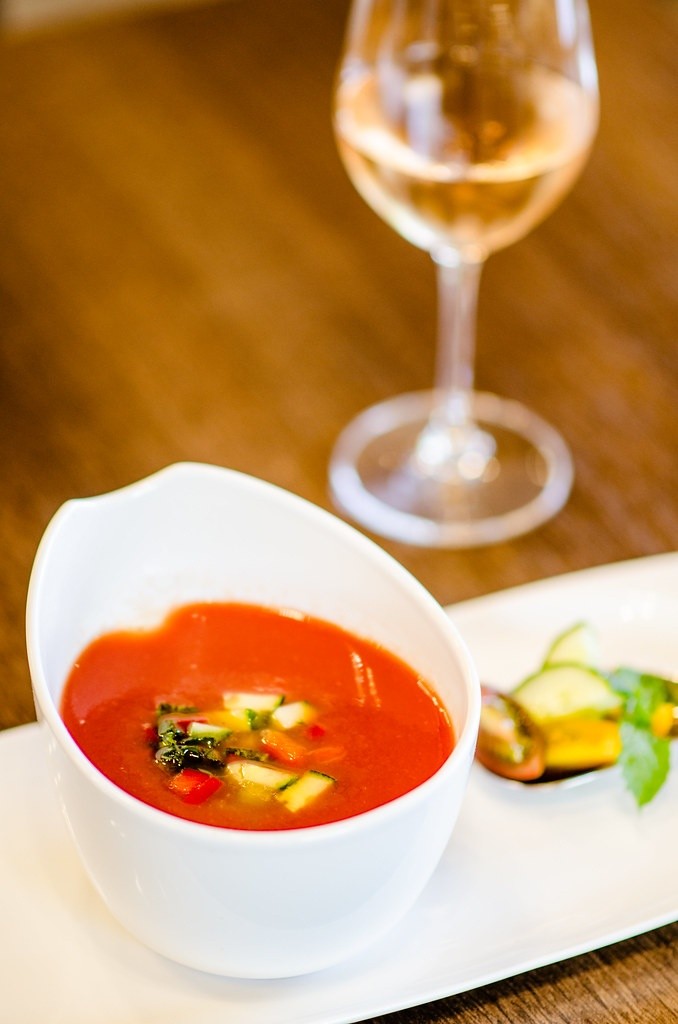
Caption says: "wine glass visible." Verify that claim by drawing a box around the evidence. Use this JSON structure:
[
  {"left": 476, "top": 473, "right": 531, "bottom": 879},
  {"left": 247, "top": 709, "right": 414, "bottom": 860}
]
[{"left": 324, "top": 0, "right": 602, "bottom": 550}]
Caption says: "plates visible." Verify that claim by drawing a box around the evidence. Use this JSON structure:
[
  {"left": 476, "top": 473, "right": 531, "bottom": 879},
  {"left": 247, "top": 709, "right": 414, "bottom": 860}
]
[{"left": 0, "top": 551, "right": 678, "bottom": 1024}]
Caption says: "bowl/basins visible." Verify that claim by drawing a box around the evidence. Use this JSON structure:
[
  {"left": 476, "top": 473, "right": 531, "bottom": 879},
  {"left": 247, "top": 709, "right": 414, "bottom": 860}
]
[{"left": 23, "top": 464, "right": 490, "bottom": 976}]
